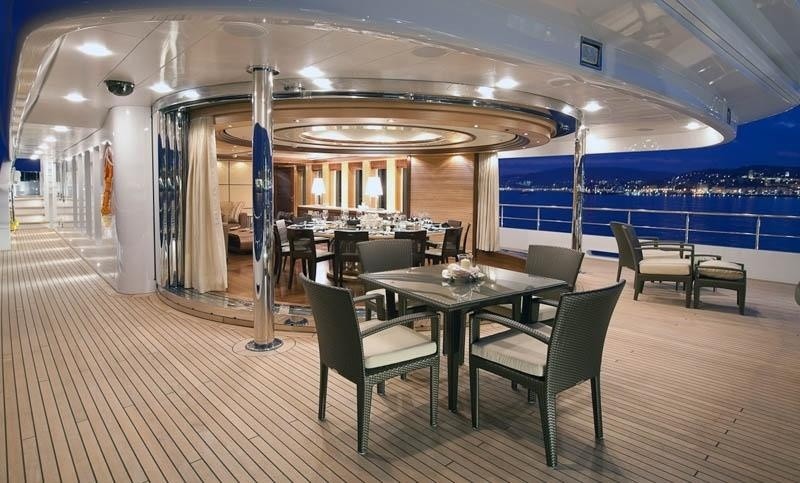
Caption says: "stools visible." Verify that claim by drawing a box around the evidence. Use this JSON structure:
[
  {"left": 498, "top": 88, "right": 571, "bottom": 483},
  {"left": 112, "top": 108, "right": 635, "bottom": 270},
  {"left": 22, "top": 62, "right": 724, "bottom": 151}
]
[
  {"left": 686, "top": 254, "right": 721, "bottom": 291},
  {"left": 694, "top": 259, "right": 746, "bottom": 315}
]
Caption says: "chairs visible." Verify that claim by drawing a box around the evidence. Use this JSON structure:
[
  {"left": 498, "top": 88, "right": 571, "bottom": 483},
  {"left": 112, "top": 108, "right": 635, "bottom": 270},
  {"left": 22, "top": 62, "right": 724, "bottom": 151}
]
[
  {"left": 473, "top": 245, "right": 584, "bottom": 391},
  {"left": 469, "top": 279, "right": 627, "bottom": 467},
  {"left": 609, "top": 221, "right": 687, "bottom": 292},
  {"left": 299, "top": 270, "right": 440, "bottom": 455},
  {"left": 355, "top": 239, "right": 445, "bottom": 377},
  {"left": 622, "top": 226, "right": 694, "bottom": 308},
  {"left": 272, "top": 214, "right": 471, "bottom": 290}
]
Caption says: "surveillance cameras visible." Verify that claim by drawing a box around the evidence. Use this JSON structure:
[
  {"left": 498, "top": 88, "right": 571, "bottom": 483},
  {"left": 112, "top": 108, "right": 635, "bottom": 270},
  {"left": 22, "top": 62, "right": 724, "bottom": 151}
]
[{"left": 104, "top": 79, "right": 135, "bottom": 96}]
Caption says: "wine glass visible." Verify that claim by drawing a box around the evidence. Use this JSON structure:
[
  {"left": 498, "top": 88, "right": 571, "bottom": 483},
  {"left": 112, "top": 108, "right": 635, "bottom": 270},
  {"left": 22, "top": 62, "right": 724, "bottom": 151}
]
[{"left": 304, "top": 210, "right": 436, "bottom": 234}]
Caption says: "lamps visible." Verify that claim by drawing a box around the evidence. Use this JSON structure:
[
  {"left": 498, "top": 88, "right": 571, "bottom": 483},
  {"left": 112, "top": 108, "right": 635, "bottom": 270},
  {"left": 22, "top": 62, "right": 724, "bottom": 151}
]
[
  {"left": 363, "top": 177, "right": 383, "bottom": 197},
  {"left": 310, "top": 178, "right": 326, "bottom": 196}
]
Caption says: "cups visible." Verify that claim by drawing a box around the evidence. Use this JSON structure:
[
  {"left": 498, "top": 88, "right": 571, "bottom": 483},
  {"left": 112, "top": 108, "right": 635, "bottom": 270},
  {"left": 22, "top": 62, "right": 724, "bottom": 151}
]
[{"left": 457, "top": 253, "right": 475, "bottom": 272}]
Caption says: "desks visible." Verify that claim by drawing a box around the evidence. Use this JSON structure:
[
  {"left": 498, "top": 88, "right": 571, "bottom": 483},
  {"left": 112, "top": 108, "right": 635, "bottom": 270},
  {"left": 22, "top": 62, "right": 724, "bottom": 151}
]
[
  {"left": 229, "top": 223, "right": 254, "bottom": 255},
  {"left": 297, "top": 204, "right": 403, "bottom": 222},
  {"left": 359, "top": 263, "right": 567, "bottom": 411}
]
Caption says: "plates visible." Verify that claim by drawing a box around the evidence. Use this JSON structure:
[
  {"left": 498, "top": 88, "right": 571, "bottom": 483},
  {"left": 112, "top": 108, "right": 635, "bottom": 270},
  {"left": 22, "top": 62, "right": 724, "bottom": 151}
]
[
  {"left": 440, "top": 264, "right": 485, "bottom": 280},
  {"left": 230, "top": 226, "right": 239, "bottom": 230},
  {"left": 239, "top": 229, "right": 254, "bottom": 232}
]
